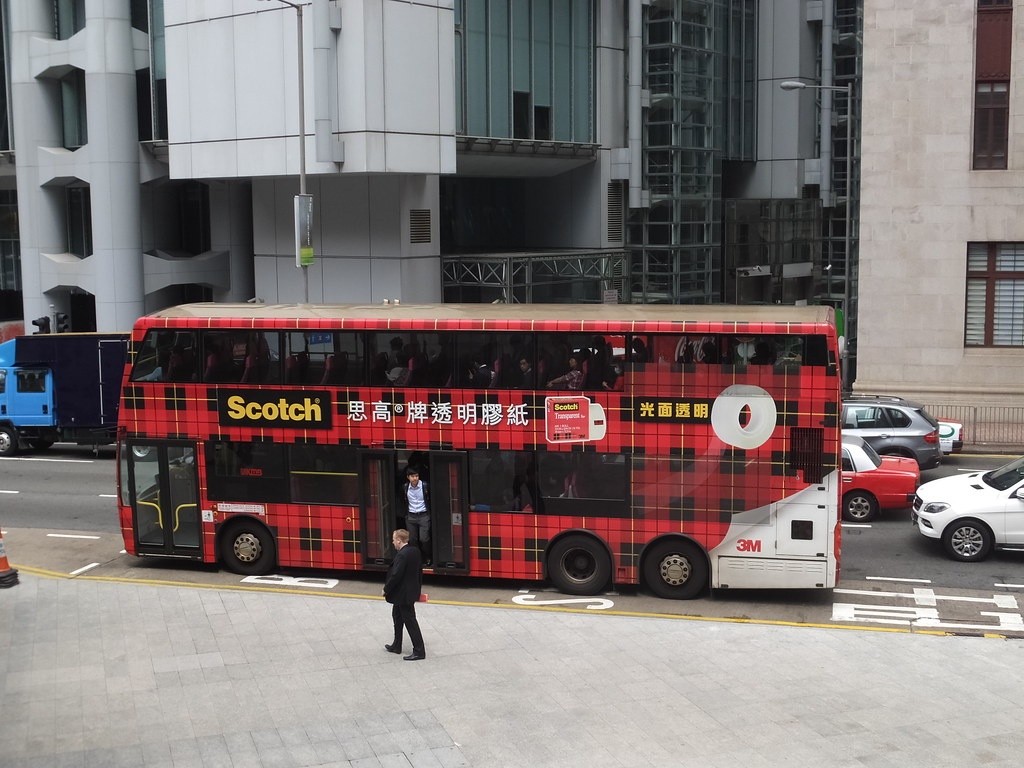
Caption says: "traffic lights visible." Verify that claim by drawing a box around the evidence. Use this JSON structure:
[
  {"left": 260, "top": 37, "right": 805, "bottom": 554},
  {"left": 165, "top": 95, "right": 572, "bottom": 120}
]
[
  {"left": 55, "top": 313, "right": 69, "bottom": 332},
  {"left": 33, "top": 318, "right": 50, "bottom": 334}
]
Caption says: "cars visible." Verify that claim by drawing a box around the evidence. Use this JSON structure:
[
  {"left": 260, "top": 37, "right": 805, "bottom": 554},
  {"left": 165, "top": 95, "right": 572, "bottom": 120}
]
[
  {"left": 840, "top": 435, "right": 921, "bottom": 521},
  {"left": 847, "top": 408, "right": 964, "bottom": 454},
  {"left": 911, "top": 454, "right": 1024, "bottom": 560}
]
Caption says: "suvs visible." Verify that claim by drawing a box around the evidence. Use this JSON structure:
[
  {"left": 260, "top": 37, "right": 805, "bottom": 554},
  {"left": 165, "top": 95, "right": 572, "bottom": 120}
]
[{"left": 840, "top": 393, "right": 942, "bottom": 471}]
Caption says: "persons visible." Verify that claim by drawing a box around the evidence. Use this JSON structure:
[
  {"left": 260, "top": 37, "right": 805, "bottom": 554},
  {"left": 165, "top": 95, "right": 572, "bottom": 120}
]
[
  {"left": 400, "top": 465, "right": 432, "bottom": 567},
  {"left": 701, "top": 341, "right": 718, "bottom": 363},
  {"left": 383, "top": 529, "right": 426, "bottom": 660},
  {"left": 293, "top": 444, "right": 391, "bottom": 507},
  {"left": 470, "top": 448, "right": 583, "bottom": 522},
  {"left": 545, "top": 345, "right": 589, "bottom": 392},
  {"left": 430, "top": 334, "right": 532, "bottom": 388},
  {"left": 152, "top": 332, "right": 190, "bottom": 380},
  {"left": 375, "top": 335, "right": 415, "bottom": 390},
  {"left": 633, "top": 337, "right": 648, "bottom": 362},
  {"left": 516, "top": 358, "right": 541, "bottom": 388}
]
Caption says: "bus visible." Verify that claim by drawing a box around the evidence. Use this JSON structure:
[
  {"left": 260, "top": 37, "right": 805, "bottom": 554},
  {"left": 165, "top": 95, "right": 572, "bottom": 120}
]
[{"left": 91, "top": 301, "right": 846, "bottom": 596}]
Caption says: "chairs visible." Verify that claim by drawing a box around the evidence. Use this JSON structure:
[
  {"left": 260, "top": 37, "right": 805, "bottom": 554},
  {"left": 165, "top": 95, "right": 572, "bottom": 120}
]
[
  {"left": 167, "top": 341, "right": 648, "bottom": 390},
  {"left": 562, "top": 460, "right": 605, "bottom": 498},
  {"left": 18, "top": 373, "right": 36, "bottom": 392}
]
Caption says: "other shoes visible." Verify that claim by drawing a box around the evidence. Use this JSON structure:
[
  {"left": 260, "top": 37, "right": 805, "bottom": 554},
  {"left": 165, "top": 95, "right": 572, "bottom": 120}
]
[{"left": 426, "top": 559, "right": 432, "bottom": 565}]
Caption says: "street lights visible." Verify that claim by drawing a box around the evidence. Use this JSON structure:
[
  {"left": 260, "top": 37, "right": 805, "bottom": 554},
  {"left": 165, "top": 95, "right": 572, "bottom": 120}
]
[{"left": 780, "top": 81, "right": 848, "bottom": 389}]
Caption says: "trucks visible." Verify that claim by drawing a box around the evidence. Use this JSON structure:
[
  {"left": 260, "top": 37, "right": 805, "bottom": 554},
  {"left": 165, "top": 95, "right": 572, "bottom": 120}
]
[{"left": 0, "top": 331, "right": 188, "bottom": 461}]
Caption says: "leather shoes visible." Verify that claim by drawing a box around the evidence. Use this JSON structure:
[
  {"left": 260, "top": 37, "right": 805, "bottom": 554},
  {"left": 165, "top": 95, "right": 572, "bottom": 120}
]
[
  {"left": 385, "top": 644, "right": 401, "bottom": 654},
  {"left": 403, "top": 654, "right": 422, "bottom": 660}
]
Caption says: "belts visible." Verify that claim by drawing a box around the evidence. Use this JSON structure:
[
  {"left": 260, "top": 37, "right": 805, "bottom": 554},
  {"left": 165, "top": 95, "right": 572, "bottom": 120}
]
[{"left": 409, "top": 511, "right": 428, "bottom": 515}]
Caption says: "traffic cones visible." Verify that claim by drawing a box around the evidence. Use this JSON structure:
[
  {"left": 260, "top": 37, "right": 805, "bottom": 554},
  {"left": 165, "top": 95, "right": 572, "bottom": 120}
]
[{"left": 0, "top": 528, "right": 19, "bottom": 590}]
